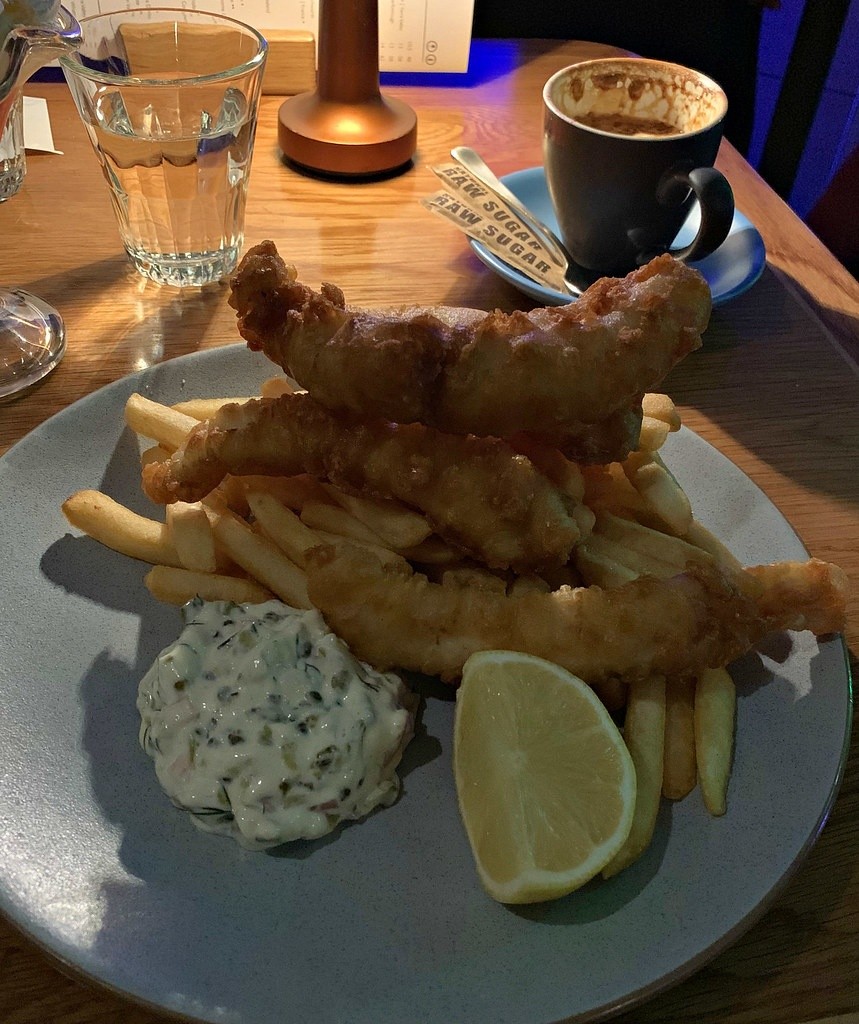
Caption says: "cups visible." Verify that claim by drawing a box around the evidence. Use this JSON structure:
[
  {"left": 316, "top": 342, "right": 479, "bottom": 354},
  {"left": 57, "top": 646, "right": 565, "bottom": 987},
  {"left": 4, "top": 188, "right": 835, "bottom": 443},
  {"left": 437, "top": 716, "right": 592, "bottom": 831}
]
[
  {"left": 0, "top": 0, "right": 86, "bottom": 200},
  {"left": 541, "top": 58, "right": 735, "bottom": 280},
  {"left": 61, "top": 8, "right": 270, "bottom": 288}
]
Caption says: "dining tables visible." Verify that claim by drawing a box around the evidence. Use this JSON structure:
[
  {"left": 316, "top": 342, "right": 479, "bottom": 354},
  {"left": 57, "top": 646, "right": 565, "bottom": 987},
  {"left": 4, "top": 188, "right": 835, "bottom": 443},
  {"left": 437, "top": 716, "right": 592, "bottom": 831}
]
[{"left": 1, "top": 37, "right": 859, "bottom": 1024}]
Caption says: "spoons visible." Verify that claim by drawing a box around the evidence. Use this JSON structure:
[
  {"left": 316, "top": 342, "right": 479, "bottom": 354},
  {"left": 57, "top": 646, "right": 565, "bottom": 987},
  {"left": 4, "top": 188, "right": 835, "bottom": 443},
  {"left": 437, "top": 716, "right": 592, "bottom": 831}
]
[{"left": 451, "top": 145, "right": 608, "bottom": 297}]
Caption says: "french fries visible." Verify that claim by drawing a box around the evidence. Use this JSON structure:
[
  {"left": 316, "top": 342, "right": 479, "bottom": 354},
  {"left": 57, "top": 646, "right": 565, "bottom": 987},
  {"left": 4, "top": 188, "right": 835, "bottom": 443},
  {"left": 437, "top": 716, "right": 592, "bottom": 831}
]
[{"left": 60, "top": 367, "right": 741, "bottom": 879}]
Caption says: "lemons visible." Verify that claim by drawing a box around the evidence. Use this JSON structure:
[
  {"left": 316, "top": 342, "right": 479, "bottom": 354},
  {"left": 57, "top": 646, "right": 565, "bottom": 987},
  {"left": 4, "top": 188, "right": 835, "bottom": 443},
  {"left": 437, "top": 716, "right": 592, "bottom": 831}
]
[{"left": 450, "top": 650, "right": 639, "bottom": 904}]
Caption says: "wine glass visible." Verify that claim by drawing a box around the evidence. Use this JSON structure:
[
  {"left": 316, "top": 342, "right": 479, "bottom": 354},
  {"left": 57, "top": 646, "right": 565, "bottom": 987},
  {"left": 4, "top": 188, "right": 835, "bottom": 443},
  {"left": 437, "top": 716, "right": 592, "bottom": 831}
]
[{"left": 0, "top": 286, "right": 68, "bottom": 400}]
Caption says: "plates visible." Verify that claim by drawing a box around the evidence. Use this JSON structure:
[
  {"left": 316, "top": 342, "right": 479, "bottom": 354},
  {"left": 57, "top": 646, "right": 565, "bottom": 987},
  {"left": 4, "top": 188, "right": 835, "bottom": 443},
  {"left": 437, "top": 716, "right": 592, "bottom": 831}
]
[
  {"left": 0, "top": 342, "right": 851, "bottom": 1024},
  {"left": 466, "top": 162, "right": 765, "bottom": 309}
]
